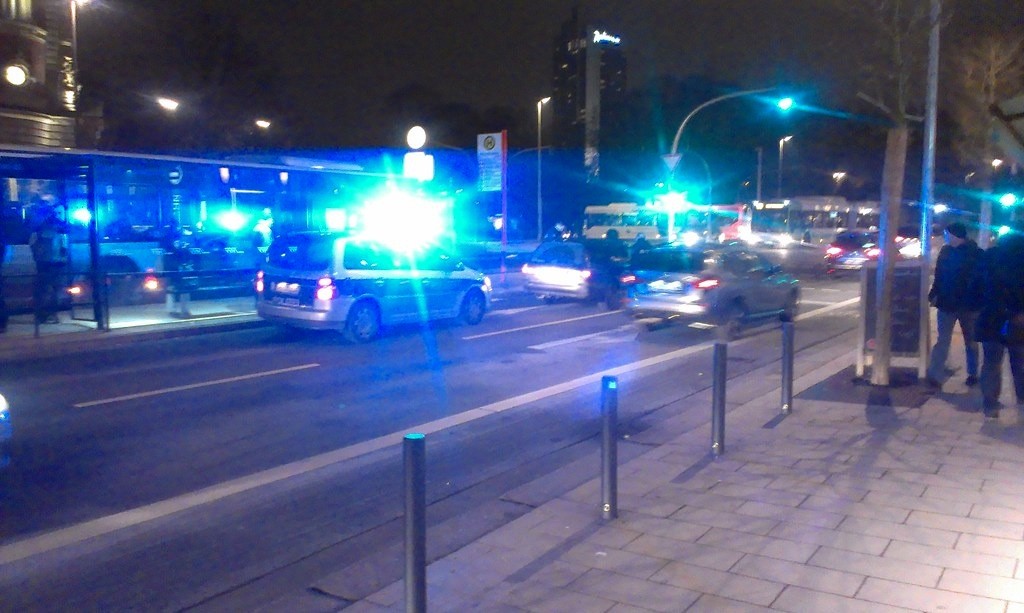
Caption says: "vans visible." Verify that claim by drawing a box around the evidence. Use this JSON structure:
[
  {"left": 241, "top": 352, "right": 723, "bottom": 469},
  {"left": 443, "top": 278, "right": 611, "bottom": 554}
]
[{"left": 254, "top": 232, "right": 493, "bottom": 344}]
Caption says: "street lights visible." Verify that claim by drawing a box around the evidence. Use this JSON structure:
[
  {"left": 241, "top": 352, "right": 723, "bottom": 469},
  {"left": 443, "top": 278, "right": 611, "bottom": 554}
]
[
  {"left": 4, "top": 0, "right": 104, "bottom": 146},
  {"left": 669, "top": 88, "right": 798, "bottom": 159},
  {"left": 778, "top": 135, "right": 794, "bottom": 196},
  {"left": 537, "top": 97, "right": 551, "bottom": 240}
]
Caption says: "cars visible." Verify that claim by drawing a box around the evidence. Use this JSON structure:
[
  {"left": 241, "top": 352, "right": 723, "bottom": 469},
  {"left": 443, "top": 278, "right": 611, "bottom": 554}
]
[
  {"left": 819, "top": 227, "right": 882, "bottom": 273},
  {"left": 521, "top": 240, "right": 630, "bottom": 303}
]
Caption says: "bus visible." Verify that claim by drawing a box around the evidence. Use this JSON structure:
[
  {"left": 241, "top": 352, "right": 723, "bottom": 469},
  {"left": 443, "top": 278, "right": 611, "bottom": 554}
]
[
  {"left": 751, "top": 193, "right": 852, "bottom": 242},
  {"left": 848, "top": 199, "right": 881, "bottom": 230},
  {"left": 584, "top": 206, "right": 753, "bottom": 243},
  {"left": 0, "top": 141, "right": 360, "bottom": 312}
]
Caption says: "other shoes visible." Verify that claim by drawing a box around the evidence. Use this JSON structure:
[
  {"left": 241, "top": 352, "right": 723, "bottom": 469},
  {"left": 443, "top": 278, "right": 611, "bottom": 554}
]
[
  {"left": 920, "top": 379, "right": 942, "bottom": 399},
  {"left": 966, "top": 375, "right": 978, "bottom": 386}
]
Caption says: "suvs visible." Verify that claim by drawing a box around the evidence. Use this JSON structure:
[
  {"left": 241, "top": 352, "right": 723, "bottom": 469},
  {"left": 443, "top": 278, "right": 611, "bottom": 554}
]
[{"left": 623, "top": 245, "right": 800, "bottom": 339}]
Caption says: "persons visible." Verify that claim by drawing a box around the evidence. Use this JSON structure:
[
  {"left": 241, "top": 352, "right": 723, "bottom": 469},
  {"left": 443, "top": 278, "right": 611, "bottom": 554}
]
[
  {"left": 0, "top": 164, "right": 890, "bottom": 339},
  {"left": 927, "top": 219, "right": 986, "bottom": 387},
  {"left": 962, "top": 221, "right": 1024, "bottom": 417}
]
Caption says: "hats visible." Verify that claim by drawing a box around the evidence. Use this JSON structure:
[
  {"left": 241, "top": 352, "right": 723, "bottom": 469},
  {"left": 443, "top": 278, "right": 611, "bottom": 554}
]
[{"left": 946, "top": 222, "right": 967, "bottom": 240}]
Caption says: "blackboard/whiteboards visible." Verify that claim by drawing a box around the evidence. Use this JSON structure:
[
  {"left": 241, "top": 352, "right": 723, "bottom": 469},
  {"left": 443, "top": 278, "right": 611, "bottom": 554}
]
[{"left": 857, "top": 259, "right": 931, "bottom": 368}]
